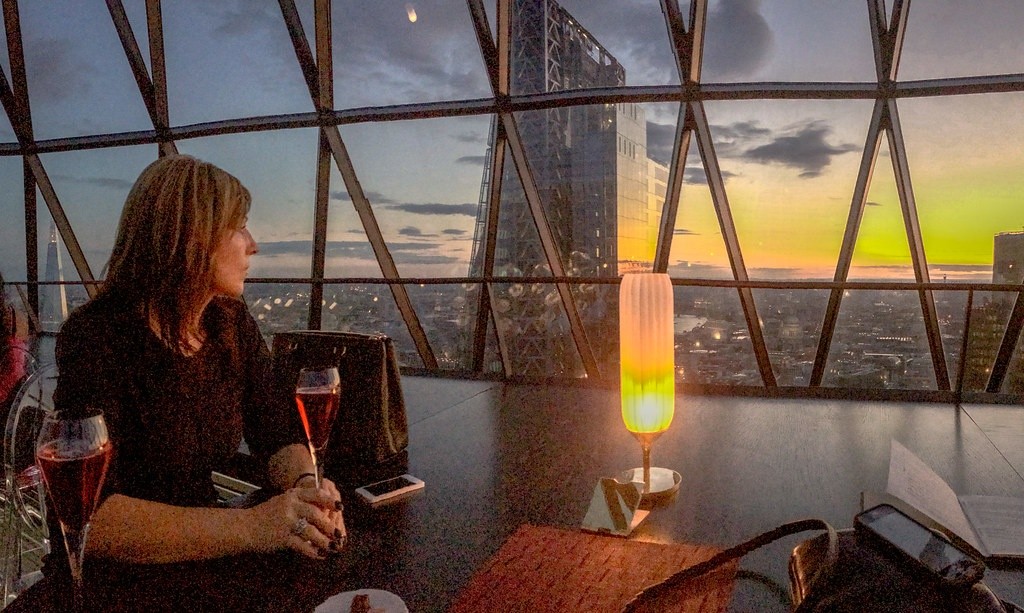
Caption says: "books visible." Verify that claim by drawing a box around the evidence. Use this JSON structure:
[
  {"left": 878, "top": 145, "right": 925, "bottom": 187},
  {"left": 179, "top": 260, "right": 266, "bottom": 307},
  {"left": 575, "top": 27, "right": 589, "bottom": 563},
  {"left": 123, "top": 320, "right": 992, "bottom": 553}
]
[{"left": 885, "top": 437, "right": 1024, "bottom": 563}]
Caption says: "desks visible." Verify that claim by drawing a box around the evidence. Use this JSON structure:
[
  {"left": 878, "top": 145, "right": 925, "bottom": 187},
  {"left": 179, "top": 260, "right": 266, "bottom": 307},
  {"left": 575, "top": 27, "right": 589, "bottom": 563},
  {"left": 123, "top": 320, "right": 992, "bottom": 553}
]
[{"left": 0, "top": 375, "right": 1024, "bottom": 613}]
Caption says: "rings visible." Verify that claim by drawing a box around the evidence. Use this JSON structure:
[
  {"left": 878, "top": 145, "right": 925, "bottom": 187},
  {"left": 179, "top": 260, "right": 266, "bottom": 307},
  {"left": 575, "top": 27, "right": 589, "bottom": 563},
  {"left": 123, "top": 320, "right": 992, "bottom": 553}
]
[{"left": 294, "top": 517, "right": 307, "bottom": 532}]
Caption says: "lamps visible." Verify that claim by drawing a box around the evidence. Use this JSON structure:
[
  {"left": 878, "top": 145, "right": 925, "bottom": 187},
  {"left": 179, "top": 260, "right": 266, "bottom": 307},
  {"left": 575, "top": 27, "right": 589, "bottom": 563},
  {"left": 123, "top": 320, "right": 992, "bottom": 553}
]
[{"left": 611, "top": 271, "right": 683, "bottom": 514}]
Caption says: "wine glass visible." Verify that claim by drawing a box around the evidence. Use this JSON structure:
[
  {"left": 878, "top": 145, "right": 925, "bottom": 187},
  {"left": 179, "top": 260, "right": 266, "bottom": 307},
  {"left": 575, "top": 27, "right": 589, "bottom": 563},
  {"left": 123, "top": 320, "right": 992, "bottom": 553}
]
[
  {"left": 35, "top": 407, "right": 113, "bottom": 613},
  {"left": 295, "top": 365, "right": 341, "bottom": 491}
]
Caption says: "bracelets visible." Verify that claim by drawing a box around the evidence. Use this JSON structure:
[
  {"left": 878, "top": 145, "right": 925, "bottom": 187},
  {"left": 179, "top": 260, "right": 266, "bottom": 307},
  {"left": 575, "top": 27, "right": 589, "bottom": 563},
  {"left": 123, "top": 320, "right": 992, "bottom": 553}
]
[{"left": 294, "top": 473, "right": 316, "bottom": 490}]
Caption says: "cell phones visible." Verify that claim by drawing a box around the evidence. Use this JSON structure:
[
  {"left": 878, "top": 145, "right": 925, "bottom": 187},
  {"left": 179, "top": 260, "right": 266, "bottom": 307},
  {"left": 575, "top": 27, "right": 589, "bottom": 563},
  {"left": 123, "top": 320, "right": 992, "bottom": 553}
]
[
  {"left": 854, "top": 502, "right": 985, "bottom": 587},
  {"left": 354, "top": 475, "right": 425, "bottom": 509}
]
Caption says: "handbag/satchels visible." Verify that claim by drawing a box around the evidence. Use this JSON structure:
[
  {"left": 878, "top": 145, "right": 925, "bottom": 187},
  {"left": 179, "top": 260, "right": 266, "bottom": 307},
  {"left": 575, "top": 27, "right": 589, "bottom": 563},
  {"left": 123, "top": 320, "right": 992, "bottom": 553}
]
[
  {"left": 270, "top": 331, "right": 409, "bottom": 466},
  {"left": 786, "top": 528, "right": 1006, "bottom": 613}
]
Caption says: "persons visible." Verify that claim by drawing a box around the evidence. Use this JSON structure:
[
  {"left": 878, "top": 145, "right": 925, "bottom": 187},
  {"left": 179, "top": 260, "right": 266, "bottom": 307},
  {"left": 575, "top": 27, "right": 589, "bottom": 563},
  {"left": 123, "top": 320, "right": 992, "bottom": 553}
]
[
  {"left": 0, "top": 276, "right": 30, "bottom": 416},
  {"left": 53, "top": 155, "right": 349, "bottom": 560}
]
